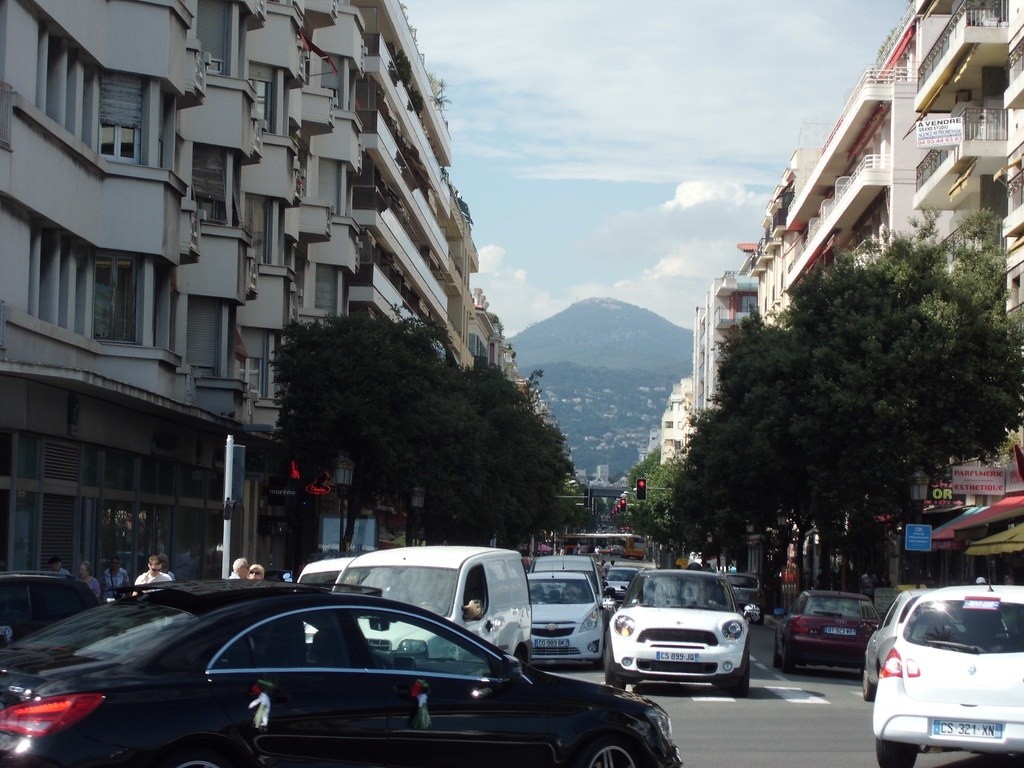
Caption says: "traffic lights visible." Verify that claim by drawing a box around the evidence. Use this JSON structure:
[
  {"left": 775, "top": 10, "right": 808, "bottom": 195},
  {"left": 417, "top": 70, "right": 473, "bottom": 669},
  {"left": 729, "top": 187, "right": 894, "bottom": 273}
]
[
  {"left": 636, "top": 478, "right": 647, "bottom": 501},
  {"left": 617, "top": 503, "right": 621, "bottom": 513},
  {"left": 610, "top": 506, "right": 618, "bottom": 517},
  {"left": 620, "top": 499, "right": 627, "bottom": 513}
]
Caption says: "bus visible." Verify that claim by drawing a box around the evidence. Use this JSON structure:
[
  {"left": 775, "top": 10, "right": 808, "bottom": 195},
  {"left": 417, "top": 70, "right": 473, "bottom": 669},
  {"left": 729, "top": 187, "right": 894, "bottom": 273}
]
[{"left": 563, "top": 533, "right": 647, "bottom": 560}]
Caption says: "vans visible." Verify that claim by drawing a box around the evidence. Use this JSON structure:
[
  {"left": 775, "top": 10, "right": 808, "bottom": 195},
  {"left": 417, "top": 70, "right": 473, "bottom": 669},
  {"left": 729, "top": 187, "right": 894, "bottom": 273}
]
[
  {"left": 527, "top": 554, "right": 616, "bottom": 630},
  {"left": 295, "top": 556, "right": 357, "bottom": 644},
  {"left": 328, "top": 546, "right": 531, "bottom": 694}
]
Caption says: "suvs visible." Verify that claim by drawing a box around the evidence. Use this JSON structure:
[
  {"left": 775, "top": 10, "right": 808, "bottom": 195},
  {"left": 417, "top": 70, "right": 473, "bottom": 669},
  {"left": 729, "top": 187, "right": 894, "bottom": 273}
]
[
  {"left": 603, "top": 566, "right": 761, "bottom": 697},
  {"left": 0, "top": 568, "right": 103, "bottom": 645}
]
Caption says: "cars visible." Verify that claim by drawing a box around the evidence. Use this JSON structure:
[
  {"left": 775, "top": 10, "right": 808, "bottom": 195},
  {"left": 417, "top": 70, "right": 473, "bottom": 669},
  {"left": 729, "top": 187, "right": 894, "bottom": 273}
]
[
  {"left": 601, "top": 566, "right": 645, "bottom": 604},
  {"left": 722, "top": 571, "right": 765, "bottom": 621},
  {"left": 0, "top": 576, "right": 687, "bottom": 768},
  {"left": 525, "top": 570, "right": 617, "bottom": 671},
  {"left": 771, "top": 589, "right": 881, "bottom": 677},
  {"left": 858, "top": 587, "right": 940, "bottom": 701},
  {"left": 873, "top": 584, "right": 1024, "bottom": 768}
]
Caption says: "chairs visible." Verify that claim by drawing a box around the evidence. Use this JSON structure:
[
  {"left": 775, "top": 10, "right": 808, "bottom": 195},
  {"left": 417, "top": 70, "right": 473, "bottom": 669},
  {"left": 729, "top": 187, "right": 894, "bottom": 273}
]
[
  {"left": 254, "top": 623, "right": 392, "bottom": 669},
  {"left": 532, "top": 584, "right": 562, "bottom": 601}
]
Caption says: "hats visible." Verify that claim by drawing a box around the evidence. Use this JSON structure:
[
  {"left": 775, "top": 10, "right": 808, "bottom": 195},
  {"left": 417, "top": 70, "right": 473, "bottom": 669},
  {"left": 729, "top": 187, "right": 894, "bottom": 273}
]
[{"left": 48, "top": 557, "right": 61, "bottom": 564}]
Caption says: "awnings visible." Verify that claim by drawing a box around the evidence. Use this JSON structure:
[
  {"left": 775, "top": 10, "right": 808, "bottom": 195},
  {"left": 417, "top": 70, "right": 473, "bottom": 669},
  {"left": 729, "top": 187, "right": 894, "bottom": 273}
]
[{"left": 928, "top": 496, "right": 1024, "bottom": 555}]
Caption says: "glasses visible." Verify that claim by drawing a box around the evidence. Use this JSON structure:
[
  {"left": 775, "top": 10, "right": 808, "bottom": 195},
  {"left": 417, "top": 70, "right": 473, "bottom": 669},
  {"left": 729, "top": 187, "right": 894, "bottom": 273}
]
[
  {"left": 250, "top": 571, "right": 260, "bottom": 574},
  {"left": 112, "top": 562, "right": 119, "bottom": 565},
  {"left": 152, "top": 568, "right": 161, "bottom": 571}
]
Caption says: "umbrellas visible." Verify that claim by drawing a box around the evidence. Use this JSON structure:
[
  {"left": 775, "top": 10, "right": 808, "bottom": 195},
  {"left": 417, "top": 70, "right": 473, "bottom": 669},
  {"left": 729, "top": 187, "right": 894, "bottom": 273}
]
[{"left": 520, "top": 543, "right": 552, "bottom": 552}]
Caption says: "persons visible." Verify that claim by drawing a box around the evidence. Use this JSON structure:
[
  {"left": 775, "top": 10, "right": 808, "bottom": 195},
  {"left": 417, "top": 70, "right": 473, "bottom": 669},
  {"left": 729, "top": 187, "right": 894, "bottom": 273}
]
[
  {"left": 562, "top": 585, "right": 579, "bottom": 603},
  {"left": 532, "top": 583, "right": 544, "bottom": 601},
  {"left": 862, "top": 566, "right": 877, "bottom": 596},
  {"left": 44, "top": 556, "right": 70, "bottom": 577},
  {"left": 554, "top": 547, "right": 565, "bottom": 555},
  {"left": 728, "top": 564, "right": 737, "bottom": 573},
  {"left": 600, "top": 560, "right": 615, "bottom": 579},
  {"left": 131, "top": 553, "right": 174, "bottom": 596},
  {"left": 229, "top": 558, "right": 248, "bottom": 579},
  {"left": 247, "top": 564, "right": 264, "bottom": 580},
  {"left": 75, "top": 560, "right": 100, "bottom": 597},
  {"left": 573, "top": 547, "right": 579, "bottom": 554},
  {"left": 703, "top": 563, "right": 712, "bottom": 571},
  {"left": 100, "top": 557, "right": 130, "bottom": 602},
  {"left": 595, "top": 545, "right": 599, "bottom": 562},
  {"left": 1003, "top": 569, "right": 1017, "bottom": 585}
]
